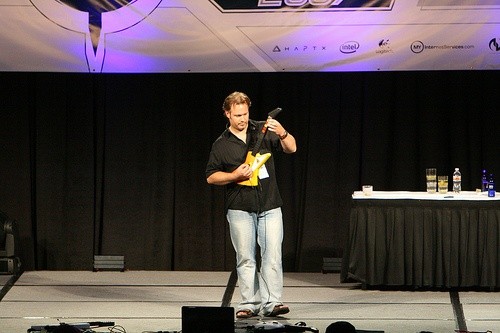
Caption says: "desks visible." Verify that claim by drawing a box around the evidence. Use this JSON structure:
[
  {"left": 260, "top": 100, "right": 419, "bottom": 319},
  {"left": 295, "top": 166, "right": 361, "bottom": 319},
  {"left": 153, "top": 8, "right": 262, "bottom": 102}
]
[{"left": 341, "top": 191, "right": 500, "bottom": 294}]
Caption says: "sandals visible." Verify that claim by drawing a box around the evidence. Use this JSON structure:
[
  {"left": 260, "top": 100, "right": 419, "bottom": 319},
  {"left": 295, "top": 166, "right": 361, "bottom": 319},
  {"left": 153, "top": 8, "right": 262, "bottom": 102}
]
[
  {"left": 236, "top": 311, "right": 258, "bottom": 318},
  {"left": 264, "top": 304, "right": 289, "bottom": 317}
]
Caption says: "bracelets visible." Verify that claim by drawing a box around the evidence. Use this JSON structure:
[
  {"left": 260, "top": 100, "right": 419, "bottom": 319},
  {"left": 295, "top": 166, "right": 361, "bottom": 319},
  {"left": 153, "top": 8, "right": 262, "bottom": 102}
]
[{"left": 279, "top": 130, "right": 289, "bottom": 140}]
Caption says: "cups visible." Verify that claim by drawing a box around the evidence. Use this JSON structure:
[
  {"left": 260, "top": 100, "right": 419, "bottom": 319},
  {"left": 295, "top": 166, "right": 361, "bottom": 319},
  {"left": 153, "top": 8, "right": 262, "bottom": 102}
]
[
  {"left": 438, "top": 176, "right": 448, "bottom": 193},
  {"left": 426, "top": 169, "right": 436, "bottom": 193},
  {"left": 362, "top": 185, "right": 373, "bottom": 196}
]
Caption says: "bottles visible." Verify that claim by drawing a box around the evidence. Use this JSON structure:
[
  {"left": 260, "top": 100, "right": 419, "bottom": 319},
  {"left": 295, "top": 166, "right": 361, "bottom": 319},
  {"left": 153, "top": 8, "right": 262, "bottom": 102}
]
[
  {"left": 488, "top": 174, "right": 495, "bottom": 197},
  {"left": 481, "top": 169, "right": 488, "bottom": 192},
  {"left": 453, "top": 168, "right": 461, "bottom": 193}
]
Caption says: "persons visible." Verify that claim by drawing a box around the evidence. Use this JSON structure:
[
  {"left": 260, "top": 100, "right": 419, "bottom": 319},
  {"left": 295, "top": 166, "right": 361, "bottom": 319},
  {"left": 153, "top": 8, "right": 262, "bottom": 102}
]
[{"left": 207, "top": 91, "right": 296, "bottom": 318}]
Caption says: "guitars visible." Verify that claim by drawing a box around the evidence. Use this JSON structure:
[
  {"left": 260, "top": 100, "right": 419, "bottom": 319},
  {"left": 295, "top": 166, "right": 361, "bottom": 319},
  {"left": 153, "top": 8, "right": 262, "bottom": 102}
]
[{"left": 235, "top": 107, "right": 283, "bottom": 186}]
